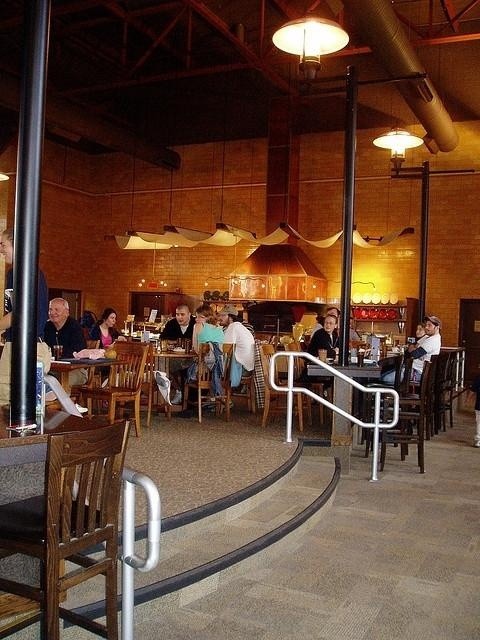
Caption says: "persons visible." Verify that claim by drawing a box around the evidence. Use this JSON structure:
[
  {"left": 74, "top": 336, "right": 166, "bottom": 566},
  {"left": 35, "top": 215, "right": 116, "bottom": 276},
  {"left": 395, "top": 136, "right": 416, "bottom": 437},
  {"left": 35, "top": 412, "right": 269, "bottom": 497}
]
[
  {"left": 159, "top": 305, "right": 197, "bottom": 418},
  {"left": 471, "top": 375, "right": 480, "bottom": 448},
  {"left": 309, "top": 307, "right": 341, "bottom": 341},
  {"left": 301, "top": 315, "right": 340, "bottom": 392},
  {"left": 0, "top": 227, "right": 50, "bottom": 434},
  {"left": 349, "top": 317, "right": 359, "bottom": 341},
  {"left": 88, "top": 308, "right": 127, "bottom": 409},
  {"left": 208, "top": 304, "right": 255, "bottom": 394},
  {"left": 379, "top": 315, "right": 442, "bottom": 384},
  {"left": 406, "top": 322, "right": 430, "bottom": 352},
  {"left": 43, "top": 298, "right": 89, "bottom": 414},
  {"left": 178, "top": 305, "right": 225, "bottom": 419}
]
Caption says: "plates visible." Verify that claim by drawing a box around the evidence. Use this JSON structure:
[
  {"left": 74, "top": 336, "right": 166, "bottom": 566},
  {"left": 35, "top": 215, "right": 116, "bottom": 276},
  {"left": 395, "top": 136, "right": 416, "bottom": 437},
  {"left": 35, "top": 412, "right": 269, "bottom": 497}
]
[{"left": 50, "top": 361, "right": 72, "bottom": 364}]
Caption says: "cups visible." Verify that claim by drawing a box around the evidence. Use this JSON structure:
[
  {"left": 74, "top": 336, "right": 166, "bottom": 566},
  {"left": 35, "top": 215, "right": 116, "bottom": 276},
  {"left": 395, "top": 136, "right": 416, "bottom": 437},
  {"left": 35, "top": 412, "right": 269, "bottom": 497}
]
[
  {"left": 137, "top": 331, "right": 142, "bottom": 338},
  {"left": 394, "top": 340, "right": 399, "bottom": 346},
  {"left": 357, "top": 352, "right": 365, "bottom": 367},
  {"left": 382, "top": 346, "right": 388, "bottom": 356},
  {"left": 318, "top": 349, "right": 328, "bottom": 363},
  {"left": 53, "top": 345, "right": 63, "bottom": 358},
  {"left": 176, "top": 338, "right": 191, "bottom": 354}
]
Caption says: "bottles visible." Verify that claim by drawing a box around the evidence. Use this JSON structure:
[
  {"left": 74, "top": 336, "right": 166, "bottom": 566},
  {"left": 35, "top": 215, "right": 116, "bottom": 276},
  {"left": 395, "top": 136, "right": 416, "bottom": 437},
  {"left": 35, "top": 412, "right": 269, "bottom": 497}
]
[{"left": 156, "top": 340, "right": 162, "bottom": 353}]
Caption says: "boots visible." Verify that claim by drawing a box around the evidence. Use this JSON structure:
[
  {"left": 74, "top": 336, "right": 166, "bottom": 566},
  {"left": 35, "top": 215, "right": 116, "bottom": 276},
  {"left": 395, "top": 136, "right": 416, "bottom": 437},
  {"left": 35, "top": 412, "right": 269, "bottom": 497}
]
[{"left": 171, "top": 389, "right": 182, "bottom": 404}]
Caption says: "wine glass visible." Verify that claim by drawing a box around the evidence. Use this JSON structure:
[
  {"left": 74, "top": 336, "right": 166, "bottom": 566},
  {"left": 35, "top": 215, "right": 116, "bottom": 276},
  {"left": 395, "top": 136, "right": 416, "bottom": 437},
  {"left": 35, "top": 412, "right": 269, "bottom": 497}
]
[{"left": 400, "top": 308, "right": 405, "bottom": 321}]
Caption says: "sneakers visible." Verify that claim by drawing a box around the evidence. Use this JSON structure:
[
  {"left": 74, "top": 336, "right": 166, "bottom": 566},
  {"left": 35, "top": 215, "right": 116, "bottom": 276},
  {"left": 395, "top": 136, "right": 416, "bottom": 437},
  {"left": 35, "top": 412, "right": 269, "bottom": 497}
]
[
  {"left": 60, "top": 403, "right": 89, "bottom": 414},
  {"left": 212, "top": 399, "right": 234, "bottom": 411}
]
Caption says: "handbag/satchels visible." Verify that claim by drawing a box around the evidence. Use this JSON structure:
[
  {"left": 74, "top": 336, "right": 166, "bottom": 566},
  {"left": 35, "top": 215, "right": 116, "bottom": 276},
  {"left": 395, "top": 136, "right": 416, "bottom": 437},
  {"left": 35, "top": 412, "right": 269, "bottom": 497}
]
[{"left": 230, "top": 355, "right": 242, "bottom": 387}]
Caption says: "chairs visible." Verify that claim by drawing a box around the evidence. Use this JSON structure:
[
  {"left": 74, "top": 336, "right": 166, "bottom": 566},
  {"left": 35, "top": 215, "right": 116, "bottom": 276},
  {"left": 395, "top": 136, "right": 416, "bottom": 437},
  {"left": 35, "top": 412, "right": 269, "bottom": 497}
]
[{"left": 0, "top": 421, "right": 135, "bottom": 640}]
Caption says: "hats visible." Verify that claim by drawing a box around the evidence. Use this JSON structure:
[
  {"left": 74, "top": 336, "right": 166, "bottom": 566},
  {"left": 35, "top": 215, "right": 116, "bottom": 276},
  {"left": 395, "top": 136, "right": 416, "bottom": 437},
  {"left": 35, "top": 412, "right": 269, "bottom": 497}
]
[
  {"left": 218, "top": 304, "right": 238, "bottom": 316},
  {"left": 424, "top": 315, "right": 442, "bottom": 330}
]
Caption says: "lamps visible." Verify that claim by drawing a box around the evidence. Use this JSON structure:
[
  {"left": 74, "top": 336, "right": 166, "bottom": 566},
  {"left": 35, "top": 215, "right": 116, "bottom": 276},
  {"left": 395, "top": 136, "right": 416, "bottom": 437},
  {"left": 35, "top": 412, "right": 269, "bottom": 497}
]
[
  {"left": 372, "top": 89, "right": 424, "bottom": 178},
  {"left": 273, "top": 2, "right": 350, "bottom": 82}
]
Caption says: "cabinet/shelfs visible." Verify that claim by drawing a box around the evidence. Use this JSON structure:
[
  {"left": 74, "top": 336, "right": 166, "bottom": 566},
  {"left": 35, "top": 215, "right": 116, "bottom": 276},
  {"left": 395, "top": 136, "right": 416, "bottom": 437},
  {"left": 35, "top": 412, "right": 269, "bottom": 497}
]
[
  {"left": 129, "top": 290, "right": 184, "bottom": 328},
  {"left": 353, "top": 296, "right": 419, "bottom": 345}
]
